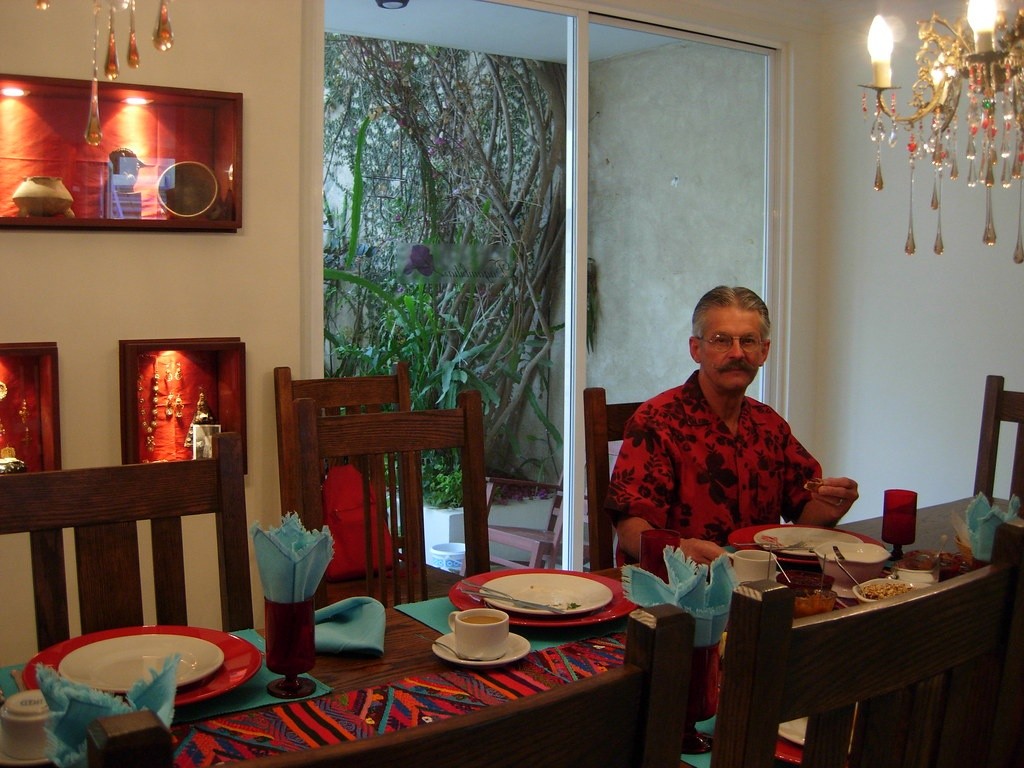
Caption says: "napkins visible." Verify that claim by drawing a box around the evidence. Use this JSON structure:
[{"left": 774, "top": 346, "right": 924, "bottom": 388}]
[
  {"left": 967, "top": 492, "right": 1022, "bottom": 562},
  {"left": 622, "top": 543, "right": 740, "bottom": 646},
  {"left": 249, "top": 512, "right": 335, "bottom": 603},
  {"left": 314, "top": 597, "right": 387, "bottom": 655},
  {"left": 33, "top": 652, "right": 181, "bottom": 768}
]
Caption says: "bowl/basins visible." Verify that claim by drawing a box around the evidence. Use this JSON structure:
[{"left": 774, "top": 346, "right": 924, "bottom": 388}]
[
  {"left": 776, "top": 570, "right": 835, "bottom": 594},
  {"left": 852, "top": 577, "right": 917, "bottom": 603},
  {"left": 813, "top": 540, "right": 892, "bottom": 599},
  {"left": 896, "top": 560, "right": 939, "bottom": 590}
]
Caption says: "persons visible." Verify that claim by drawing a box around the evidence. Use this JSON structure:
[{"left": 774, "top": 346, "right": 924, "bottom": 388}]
[
  {"left": 196, "top": 393, "right": 216, "bottom": 425},
  {"left": 607, "top": 283, "right": 860, "bottom": 572}
]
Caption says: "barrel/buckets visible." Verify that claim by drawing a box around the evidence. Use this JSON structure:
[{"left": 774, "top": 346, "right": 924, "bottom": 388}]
[{"left": 430, "top": 543, "right": 465, "bottom": 575}]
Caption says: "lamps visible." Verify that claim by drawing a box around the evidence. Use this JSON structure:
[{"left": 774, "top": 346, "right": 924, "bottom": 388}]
[{"left": 856, "top": 1, "right": 1024, "bottom": 264}]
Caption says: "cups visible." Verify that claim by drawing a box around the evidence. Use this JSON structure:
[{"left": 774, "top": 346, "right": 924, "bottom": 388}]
[
  {"left": 0, "top": 690, "right": 52, "bottom": 760},
  {"left": 726, "top": 549, "right": 778, "bottom": 583},
  {"left": 448, "top": 608, "right": 510, "bottom": 661}
]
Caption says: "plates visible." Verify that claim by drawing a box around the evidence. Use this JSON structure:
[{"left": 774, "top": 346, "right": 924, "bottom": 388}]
[
  {"left": 478, "top": 573, "right": 614, "bottom": 614},
  {"left": 727, "top": 524, "right": 885, "bottom": 564},
  {"left": 57, "top": 634, "right": 224, "bottom": 693},
  {"left": 432, "top": 631, "right": 531, "bottom": 669},
  {"left": 449, "top": 568, "right": 637, "bottom": 627},
  {"left": 753, "top": 527, "right": 864, "bottom": 556},
  {"left": 22, "top": 626, "right": 262, "bottom": 706}
]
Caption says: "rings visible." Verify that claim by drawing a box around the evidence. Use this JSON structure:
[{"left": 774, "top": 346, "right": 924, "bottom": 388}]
[{"left": 838, "top": 498, "right": 842, "bottom": 506}]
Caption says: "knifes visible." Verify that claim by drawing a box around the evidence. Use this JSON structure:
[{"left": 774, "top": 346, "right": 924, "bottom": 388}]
[{"left": 462, "top": 590, "right": 566, "bottom": 615}]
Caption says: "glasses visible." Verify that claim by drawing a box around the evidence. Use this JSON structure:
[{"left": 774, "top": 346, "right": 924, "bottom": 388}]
[{"left": 696, "top": 332, "right": 764, "bottom": 354}]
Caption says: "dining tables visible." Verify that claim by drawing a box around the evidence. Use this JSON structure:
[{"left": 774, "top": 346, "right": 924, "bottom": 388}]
[{"left": 0, "top": 498, "right": 1024, "bottom": 768}]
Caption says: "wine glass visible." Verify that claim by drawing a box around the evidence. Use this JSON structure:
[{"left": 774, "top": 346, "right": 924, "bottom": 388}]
[
  {"left": 881, "top": 489, "right": 918, "bottom": 568},
  {"left": 262, "top": 596, "right": 317, "bottom": 700}
]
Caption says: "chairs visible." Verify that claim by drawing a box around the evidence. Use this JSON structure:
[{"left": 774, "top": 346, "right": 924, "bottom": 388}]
[
  {"left": 1, "top": 433, "right": 254, "bottom": 654},
  {"left": 87, "top": 604, "right": 694, "bottom": 768},
  {"left": 273, "top": 361, "right": 490, "bottom": 607},
  {"left": 711, "top": 516, "right": 1024, "bottom": 768},
  {"left": 583, "top": 387, "right": 644, "bottom": 571},
  {"left": 486, "top": 470, "right": 615, "bottom": 571},
  {"left": 974, "top": 376, "right": 1024, "bottom": 503}
]
